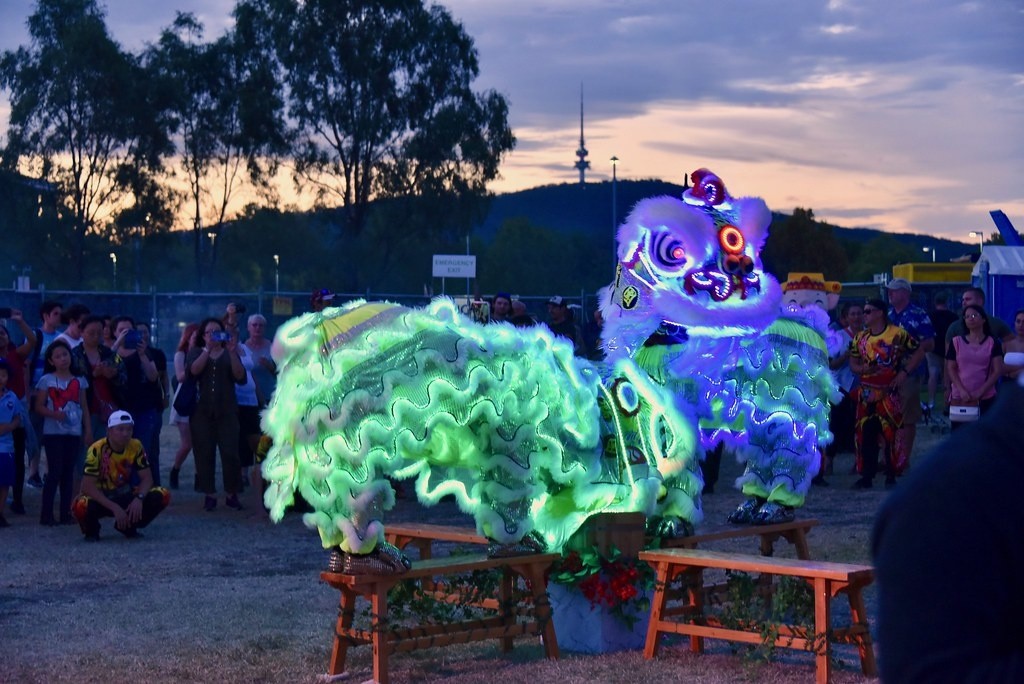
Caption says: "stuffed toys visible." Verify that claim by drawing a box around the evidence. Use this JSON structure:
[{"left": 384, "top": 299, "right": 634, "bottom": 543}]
[
  {"left": 595, "top": 170, "right": 839, "bottom": 541},
  {"left": 260, "top": 299, "right": 704, "bottom": 575}
]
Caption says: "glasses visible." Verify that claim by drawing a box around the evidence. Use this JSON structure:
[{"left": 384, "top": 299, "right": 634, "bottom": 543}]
[
  {"left": 965, "top": 313, "right": 978, "bottom": 319},
  {"left": 252, "top": 323, "right": 264, "bottom": 328},
  {"left": 863, "top": 308, "right": 871, "bottom": 314},
  {"left": 497, "top": 292, "right": 511, "bottom": 298}
]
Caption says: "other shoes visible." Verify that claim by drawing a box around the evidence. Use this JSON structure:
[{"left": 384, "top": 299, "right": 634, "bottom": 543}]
[
  {"left": 813, "top": 475, "right": 829, "bottom": 487},
  {"left": 825, "top": 463, "right": 834, "bottom": 475},
  {"left": 39, "top": 510, "right": 60, "bottom": 526},
  {"left": 84, "top": 528, "right": 101, "bottom": 542},
  {"left": 203, "top": 495, "right": 218, "bottom": 512},
  {"left": 225, "top": 495, "right": 241, "bottom": 511},
  {"left": 0, "top": 512, "right": 12, "bottom": 528},
  {"left": 10, "top": 501, "right": 26, "bottom": 516},
  {"left": 242, "top": 474, "right": 251, "bottom": 487},
  {"left": 59, "top": 512, "right": 77, "bottom": 525},
  {"left": 169, "top": 470, "right": 179, "bottom": 490},
  {"left": 26, "top": 473, "right": 46, "bottom": 488},
  {"left": 884, "top": 470, "right": 897, "bottom": 487},
  {"left": 853, "top": 478, "right": 873, "bottom": 489},
  {"left": 114, "top": 520, "right": 145, "bottom": 539}
]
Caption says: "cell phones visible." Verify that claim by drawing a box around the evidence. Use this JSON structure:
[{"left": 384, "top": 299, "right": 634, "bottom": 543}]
[
  {"left": 124, "top": 330, "right": 143, "bottom": 349},
  {"left": 211, "top": 330, "right": 232, "bottom": 342},
  {"left": 0, "top": 308, "right": 12, "bottom": 318}
]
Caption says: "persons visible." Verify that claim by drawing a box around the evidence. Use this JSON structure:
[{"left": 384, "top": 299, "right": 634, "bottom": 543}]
[
  {"left": 73, "top": 410, "right": 171, "bottom": 541},
  {"left": 870, "top": 384, "right": 1024, "bottom": 684},
  {"left": 945, "top": 289, "right": 1024, "bottom": 433},
  {"left": 491, "top": 293, "right": 603, "bottom": 360},
  {"left": 812, "top": 279, "right": 935, "bottom": 488},
  {"left": 927, "top": 297, "right": 959, "bottom": 409},
  {"left": 169, "top": 290, "right": 333, "bottom": 512},
  {"left": 0, "top": 302, "right": 170, "bottom": 527}
]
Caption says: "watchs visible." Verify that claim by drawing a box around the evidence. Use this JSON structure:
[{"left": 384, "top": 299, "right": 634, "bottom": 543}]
[{"left": 136, "top": 493, "right": 145, "bottom": 501}]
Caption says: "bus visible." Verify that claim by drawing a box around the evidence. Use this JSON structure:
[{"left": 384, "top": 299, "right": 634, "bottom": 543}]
[
  {"left": 892, "top": 264, "right": 974, "bottom": 283},
  {"left": 829, "top": 282, "right": 888, "bottom": 321}
]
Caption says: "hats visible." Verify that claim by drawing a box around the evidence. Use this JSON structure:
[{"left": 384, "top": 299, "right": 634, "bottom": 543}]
[
  {"left": 885, "top": 277, "right": 912, "bottom": 293},
  {"left": 108, "top": 410, "right": 134, "bottom": 428},
  {"left": 545, "top": 295, "right": 567, "bottom": 308},
  {"left": 865, "top": 299, "right": 888, "bottom": 317}
]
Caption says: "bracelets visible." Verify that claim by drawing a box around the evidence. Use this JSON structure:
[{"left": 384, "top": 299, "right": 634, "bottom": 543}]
[
  {"left": 902, "top": 368, "right": 910, "bottom": 377},
  {"left": 204, "top": 347, "right": 210, "bottom": 352},
  {"left": 227, "top": 321, "right": 235, "bottom": 326}
]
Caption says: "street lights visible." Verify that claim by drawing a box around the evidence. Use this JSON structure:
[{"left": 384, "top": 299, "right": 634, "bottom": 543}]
[
  {"left": 110, "top": 251, "right": 117, "bottom": 294},
  {"left": 207, "top": 233, "right": 215, "bottom": 267},
  {"left": 611, "top": 156, "right": 619, "bottom": 278},
  {"left": 969, "top": 231, "right": 983, "bottom": 254},
  {"left": 274, "top": 255, "right": 279, "bottom": 296},
  {"left": 924, "top": 247, "right": 935, "bottom": 262}
]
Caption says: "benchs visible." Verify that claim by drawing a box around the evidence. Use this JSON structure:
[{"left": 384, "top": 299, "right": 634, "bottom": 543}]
[
  {"left": 320, "top": 549, "right": 580, "bottom": 684},
  {"left": 638, "top": 548, "right": 875, "bottom": 684},
  {"left": 382, "top": 522, "right": 533, "bottom": 610},
  {"left": 666, "top": 517, "right": 818, "bottom": 625}
]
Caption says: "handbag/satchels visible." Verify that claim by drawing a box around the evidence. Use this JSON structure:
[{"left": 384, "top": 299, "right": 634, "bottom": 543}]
[
  {"left": 947, "top": 397, "right": 981, "bottom": 422},
  {"left": 172, "top": 374, "right": 196, "bottom": 417},
  {"left": 172, "top": 350, "right": 188, "bottom": 392}
]
